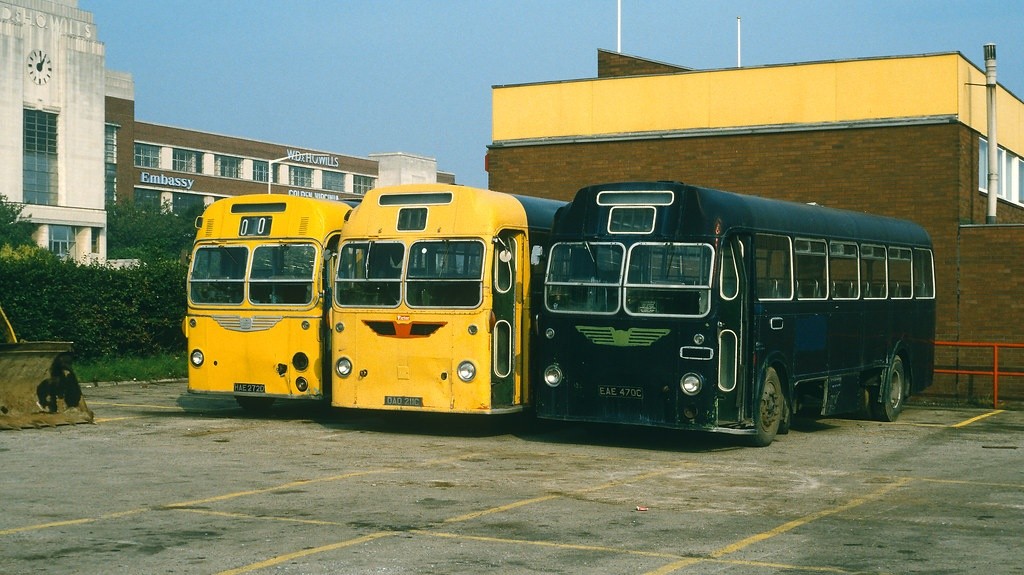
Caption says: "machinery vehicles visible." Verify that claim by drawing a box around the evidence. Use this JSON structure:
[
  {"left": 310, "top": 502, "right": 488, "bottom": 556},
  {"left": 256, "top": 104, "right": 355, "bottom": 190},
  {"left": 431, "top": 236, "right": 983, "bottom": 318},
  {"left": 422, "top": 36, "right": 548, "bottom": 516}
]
[{"left": 0, "top": 306, "right": 94, "bottom": 430}]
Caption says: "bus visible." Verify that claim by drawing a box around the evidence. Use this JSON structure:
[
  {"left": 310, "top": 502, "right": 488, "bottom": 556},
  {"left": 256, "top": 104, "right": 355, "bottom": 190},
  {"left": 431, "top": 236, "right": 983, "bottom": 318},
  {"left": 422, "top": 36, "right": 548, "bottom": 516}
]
[
  {"left": 182, "top": 194, "right": 366, "bottom": 408},
  {"left": 528, "top": 178, "right": 938, "bottom": 447},
  {"left": 329, "top": 181, "right": 573, "bottom": 414}
]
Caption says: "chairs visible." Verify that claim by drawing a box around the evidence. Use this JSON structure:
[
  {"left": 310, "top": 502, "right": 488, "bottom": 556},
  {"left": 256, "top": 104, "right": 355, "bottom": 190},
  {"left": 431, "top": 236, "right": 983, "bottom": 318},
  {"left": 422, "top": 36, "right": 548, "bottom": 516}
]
[{"left": 757, "top": 278, "right": 925, "bottom": 298}]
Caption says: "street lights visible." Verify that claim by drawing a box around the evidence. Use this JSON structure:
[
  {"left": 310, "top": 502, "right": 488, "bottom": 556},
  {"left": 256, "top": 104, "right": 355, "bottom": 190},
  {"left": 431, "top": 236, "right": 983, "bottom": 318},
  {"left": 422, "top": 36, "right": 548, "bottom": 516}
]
[{"left": 268, "top": 152, "right": 330, "bottom": 191}]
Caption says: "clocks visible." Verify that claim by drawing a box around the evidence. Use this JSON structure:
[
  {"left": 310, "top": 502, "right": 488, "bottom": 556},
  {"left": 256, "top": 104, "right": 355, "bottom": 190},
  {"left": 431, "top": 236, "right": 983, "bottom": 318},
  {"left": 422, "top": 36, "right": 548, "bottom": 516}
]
[{"left": 27, "top": 49, "right": 53, "bottom": 85}]
